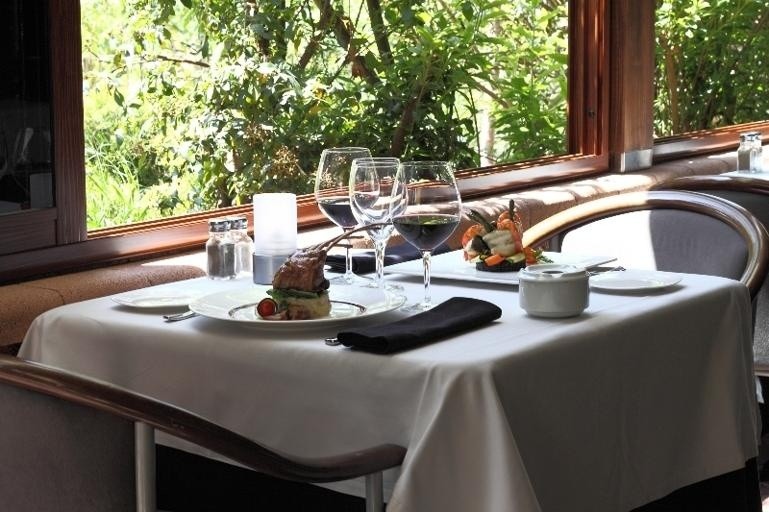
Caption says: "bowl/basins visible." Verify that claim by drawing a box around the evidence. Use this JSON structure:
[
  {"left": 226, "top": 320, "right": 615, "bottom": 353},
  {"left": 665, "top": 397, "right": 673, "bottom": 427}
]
[{"left": 515, "top": 260, "right": 595, "bottom": 322}]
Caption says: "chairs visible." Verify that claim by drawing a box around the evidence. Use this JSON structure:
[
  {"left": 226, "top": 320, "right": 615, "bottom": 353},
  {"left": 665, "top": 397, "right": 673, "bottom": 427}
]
[
  {"left": 0, "top": 352, "right": 409, "bottom": 512},
  {"left": 645, "top": 174, "right": 769, "bottom": 379},
  {"left": 521, "top": 188, "right": 768, "bottom": 344}
]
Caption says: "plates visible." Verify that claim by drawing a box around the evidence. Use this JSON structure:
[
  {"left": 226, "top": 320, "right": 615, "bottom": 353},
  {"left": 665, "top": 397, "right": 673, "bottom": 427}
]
[
  {"left": 108, "top": 271, "right": 206, "bottom": 311},
  {"left": 377, "top": 245, "right": 617, "bottom": 290},
  {"left": 587, "top": 266, "right": 685, "bottom": 293},
  {"left": 190, "top": 286, "right": 423, "bottom": 326}
]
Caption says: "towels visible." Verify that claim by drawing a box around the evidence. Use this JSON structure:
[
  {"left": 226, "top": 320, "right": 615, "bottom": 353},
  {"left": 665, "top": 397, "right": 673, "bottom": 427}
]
[
  {"left": 325, "top": 235, "right": 450, "bottom": 272},
  {"left": 337, "top": 296, "right": 501, "bottom": 354}
]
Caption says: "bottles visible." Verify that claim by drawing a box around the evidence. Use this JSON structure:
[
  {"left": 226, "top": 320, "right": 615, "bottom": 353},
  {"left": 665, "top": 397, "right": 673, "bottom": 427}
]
[
  {"left": 750, "top": 132, "right": 763, "bottom": 173},
  {"left": 205, "top": 216, "right": 233, "bottom": 280},
  {"left": 737, "top": 133, "right": 757, "bottom": 173},
  {"left": 228, "top": 216, "right": 254, "bottom": 279}
]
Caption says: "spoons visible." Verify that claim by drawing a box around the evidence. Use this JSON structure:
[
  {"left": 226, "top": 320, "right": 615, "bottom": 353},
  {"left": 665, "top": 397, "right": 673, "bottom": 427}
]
[{"left": 561, "top": 257, "right": 622, "bottom": 278}]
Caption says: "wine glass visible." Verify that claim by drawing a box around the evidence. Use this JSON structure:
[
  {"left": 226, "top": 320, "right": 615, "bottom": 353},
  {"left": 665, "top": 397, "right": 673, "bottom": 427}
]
[
  {"left": 388, "top": 160, "right": 463, "bottom": 316},
  {"left": 348, "top": 158, "right": 408, "bottom": 295},
  {"left": 314, "top": 146, "right": 375, "bottom": 285}
]
